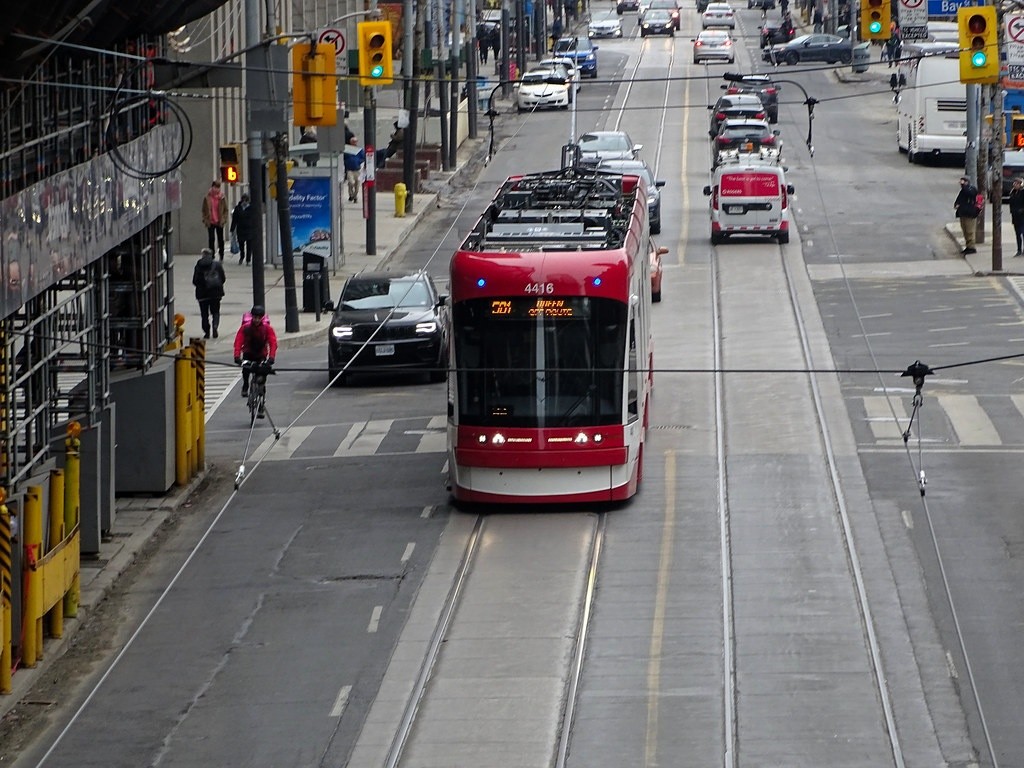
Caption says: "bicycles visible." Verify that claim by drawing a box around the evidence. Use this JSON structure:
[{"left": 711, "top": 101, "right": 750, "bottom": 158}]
[{"left": 237, "top": 359, "right": 273, "bottom": 428}]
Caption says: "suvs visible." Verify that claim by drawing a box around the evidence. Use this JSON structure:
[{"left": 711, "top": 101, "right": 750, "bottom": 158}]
[
  {"left": 323, "top": 272, "right": 451, "bottom": 389},
  {"left": 708, "top": 117, "right": 781, "bottom": 172},
  {"left": 720, "top": 73, "right": 782, "bottom": 124},
  {"left": 595, "top": 160, "right": 665, "bottom": 234}
]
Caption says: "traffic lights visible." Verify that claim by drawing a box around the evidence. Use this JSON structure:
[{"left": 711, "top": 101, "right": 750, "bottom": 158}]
[
  {"left": 357, "top": 22, "right": 393, "bottom": 87},
  {"left": 268, "top": 159, "right": 294, "bottom": 200},
  {"left": 1010, "top": 114, "right": 1024, "bottom": 148},
  {"left": 983, "top": 114, "right": 1006, "bottom": 145},
  {"left": 860, "top": 0, "right": 891, "bottom": 41},
  {"left": 218, "top": 143, "right": 241, "bottom": 184},
  {"left": 958, "top": 6, "right": 999, "bottom": 81}
]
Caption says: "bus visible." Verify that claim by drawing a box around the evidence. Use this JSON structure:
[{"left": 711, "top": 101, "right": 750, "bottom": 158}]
[{"left": 445, "top": 37, "right": 654, "bottom": 504}]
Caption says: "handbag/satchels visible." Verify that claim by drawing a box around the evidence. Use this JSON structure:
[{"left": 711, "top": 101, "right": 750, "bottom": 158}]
[{"left": 230, "top": 232, "right": 239, "bottom": 254}]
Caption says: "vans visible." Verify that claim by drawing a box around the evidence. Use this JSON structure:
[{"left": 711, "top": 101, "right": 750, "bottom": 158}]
[
  {"left": 890, "top": 20, "right": 967, "bottom": 165},
  {"left": 703, "top": 148, "right": 795, "bottom": 244}
]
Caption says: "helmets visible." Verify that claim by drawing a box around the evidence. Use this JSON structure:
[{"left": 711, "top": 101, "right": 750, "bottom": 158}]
[{"left": 252, "top": 303, "right": 265, "bottom": 318}]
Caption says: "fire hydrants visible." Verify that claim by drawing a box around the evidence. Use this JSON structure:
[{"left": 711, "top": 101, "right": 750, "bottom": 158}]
[{"left": 394, "top": 182, "right": 407, "bottom": 218}]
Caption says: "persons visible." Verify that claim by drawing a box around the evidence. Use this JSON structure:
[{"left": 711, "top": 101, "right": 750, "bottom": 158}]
[
  {"left": 299, "top": 127, "right": 320, "bottom": 167},
  {"left": 234, "top": 307, "right": 278, "bottom": 419},
  {"left": 780, "top": 15, "right": 792, "bottom": 42},
  {"left": 492, "top": 28, "right": 501, "bottom": 61},
  {"left": 193, "top": 248, "right": 226, "bottom": 339},
  {"left": 1009, "top": 178, "right": 1024, "bottom": 256},
  {"left": 489, "top": 25, "right": 499, "bottom": 49},
  {"left": 345, "top": 123, "right": 355, "bottom": 179},
  {"left": 344, "top": 137, "right": 365, "bottom": 202},
  {"left": 778, "top": 0, "right": 789, "bottom": 17},
  {"left": 230, "top": 193, "right": 252, "bottom": 266},
  {"left": 838, "top": 4, "right": 852, "bottom": 39},
  {"left": 810, "top": 0, "right": 823, "bottom": 25},
  {"left": 954, "top": 176, "right": 982, "bottom": 254},
  {"left": 386, "top": 120, "right": 404, "bottom": 158},
  {"left": 886, "top": 32, "right": 900, "bottom": 68},
  {"left": 201, "top": 180, "right": 228, "bottom": 264},
  {"left": 477, "top": 24, "right": 491, "bottom": 65},
  {"left": 787, "top": 11, "right": 792, "bottom": 28}
]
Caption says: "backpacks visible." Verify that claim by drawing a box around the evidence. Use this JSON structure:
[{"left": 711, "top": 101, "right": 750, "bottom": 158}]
[
  {"left": 242, "top": 312, "right": 270, "bottom": 325},
  {"left": 975, "top": 194, "right": 985, "bottom": 210},
  {"left": 199, "top": 263, "right": 221, "bottom": 288}
]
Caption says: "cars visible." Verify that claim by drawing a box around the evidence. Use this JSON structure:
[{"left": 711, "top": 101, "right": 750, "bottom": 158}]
[
  {"left": 987, "top": 151, "right": 1024, "bottom": 203},
  {"left": 760, "top": 33, "right": 853, "bottom": 67},
  {"left": 690, "top": 0, "right": 739, "bottom": 64},
  {"left": 570, "top": 132, "right": 644, "bottom": 169},
  {"left": 746, "top": 0, "right": 797, "bottom": 49},
  {"left": 707, "top": 93, "right": 771, "bottom": 141},
  {"left": 586, "top": 0, "right": 683, "bottom": 40},
  {"left": 512, "top": 36, "right": 600, "bottom": 113},
  {"left": 648, "top": 235, "right": 669, "bottom": 303}
]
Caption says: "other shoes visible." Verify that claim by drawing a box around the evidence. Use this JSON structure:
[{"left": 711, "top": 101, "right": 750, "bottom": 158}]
[
  {"left": 241, "top": 384, "right": 249, "bottom": 396},
  {"left": 239, "top": 257, "right": 244, "bottom": 265},
  {"left": 258, "top": 408, "right": 265, "bottom": 418},
  {"left": 213, "top": 326, "right": 218, "bottom": 338},
  {"left": 961, "top": 247, "right": 976, "bottom": 255},
  {"left": 203, "top": 332, "right": 209, "bottom": 338},
  {"left": 349, "top": 197, "right": 357, "bottom": 204},
  {"left": 1014, "top": 252, "right": 1022, "bottom": 258}
]
